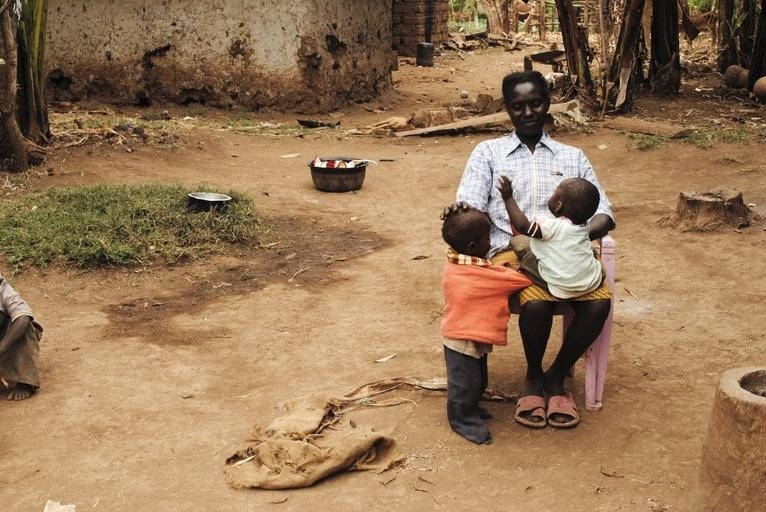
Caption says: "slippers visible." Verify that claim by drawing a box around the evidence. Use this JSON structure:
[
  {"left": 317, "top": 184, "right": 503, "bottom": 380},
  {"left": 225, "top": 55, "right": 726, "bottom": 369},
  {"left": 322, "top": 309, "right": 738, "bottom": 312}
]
[
  {"left": 546, "top": 389, "right": 580, "bottom": 428},
  {"left": 514, "top": 389, "right": 546, "bottom": 428}
]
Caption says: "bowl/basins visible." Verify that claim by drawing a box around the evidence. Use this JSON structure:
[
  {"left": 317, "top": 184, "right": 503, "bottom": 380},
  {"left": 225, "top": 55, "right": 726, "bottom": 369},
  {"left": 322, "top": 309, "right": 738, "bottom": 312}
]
[
  {"left": 306, "top": 158, "right": 368, "bottom": 191},
  {"left": 186, "top": 192, "right": 231, "bottom": 212}
]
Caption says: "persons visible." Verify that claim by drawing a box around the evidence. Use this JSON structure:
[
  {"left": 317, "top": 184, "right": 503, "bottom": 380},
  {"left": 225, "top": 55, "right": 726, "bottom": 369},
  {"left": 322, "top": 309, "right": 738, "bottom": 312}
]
[
  {"left": 0, "top": 274, "right": 43, "bottom": 400},
  {"left": 441, "top": 207, "right": 535, "bottom": 445},
  {"left": 495, "top": 174, "right": 606, "bottom": 300},
  {"left": 440, "top": 71, "right": 616, "bottom": 427}
]
